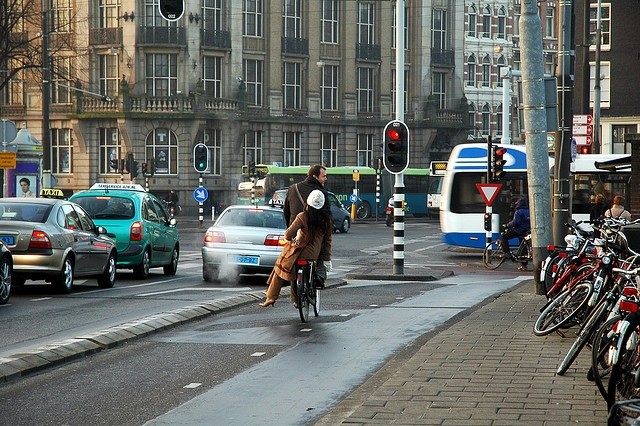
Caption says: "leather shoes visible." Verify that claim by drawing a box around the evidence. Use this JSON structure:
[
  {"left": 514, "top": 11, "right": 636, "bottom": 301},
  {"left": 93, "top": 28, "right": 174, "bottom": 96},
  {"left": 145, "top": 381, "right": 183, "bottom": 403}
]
[{"left": 315, "top": 275, "right": 324, "bottom": 290}]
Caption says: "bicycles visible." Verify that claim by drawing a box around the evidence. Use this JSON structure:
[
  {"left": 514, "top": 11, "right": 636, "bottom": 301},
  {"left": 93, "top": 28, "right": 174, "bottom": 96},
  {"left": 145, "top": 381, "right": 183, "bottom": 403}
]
[
  {"left": 296, "top": 259, "right": 321, "bottom": 323},
  {"left": 534, "top": 212, "right": 640, "bottom": 422},
  {"left": 482, "top": 224, "right": 531, "bottom": 270}
]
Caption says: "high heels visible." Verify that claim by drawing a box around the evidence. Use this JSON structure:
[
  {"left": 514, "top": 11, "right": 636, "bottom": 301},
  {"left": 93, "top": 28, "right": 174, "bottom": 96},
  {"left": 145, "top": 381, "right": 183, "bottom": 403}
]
[{"left": 259, "top": 298, "right": 275, "bottom": 307}]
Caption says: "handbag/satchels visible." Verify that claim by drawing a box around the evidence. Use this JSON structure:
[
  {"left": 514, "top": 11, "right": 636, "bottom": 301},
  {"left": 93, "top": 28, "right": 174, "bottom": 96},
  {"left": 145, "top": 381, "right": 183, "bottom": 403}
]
[{"left": 276, "top": 241, "right": 303, "bottom": 273}]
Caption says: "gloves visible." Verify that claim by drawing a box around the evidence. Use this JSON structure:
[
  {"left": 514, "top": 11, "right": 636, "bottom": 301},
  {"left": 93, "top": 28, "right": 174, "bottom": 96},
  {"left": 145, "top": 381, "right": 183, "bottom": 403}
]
[{"left": 323, "top": 260, "right": 333, "bottom": 272}]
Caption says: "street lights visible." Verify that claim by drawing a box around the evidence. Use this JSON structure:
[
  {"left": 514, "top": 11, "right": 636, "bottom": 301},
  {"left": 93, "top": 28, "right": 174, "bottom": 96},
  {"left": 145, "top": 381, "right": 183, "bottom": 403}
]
[{"left": 42, "top": 81, "right": 112, "bottom": 187}]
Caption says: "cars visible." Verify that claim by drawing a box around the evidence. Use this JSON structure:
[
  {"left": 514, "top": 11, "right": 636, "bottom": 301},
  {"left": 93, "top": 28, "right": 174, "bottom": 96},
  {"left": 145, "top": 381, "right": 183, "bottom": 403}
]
[
  {"left": 0, "top": 188, "right": 118, "bottom": 294},
  {"left": 201, "top": 199, "right": 288, "bottom": 282},
  {"left": 68, "top": 182, "right": 180, "bottom": 279},
  {"left": 0, "top": 239, "right": 14, "bottom": 304}
]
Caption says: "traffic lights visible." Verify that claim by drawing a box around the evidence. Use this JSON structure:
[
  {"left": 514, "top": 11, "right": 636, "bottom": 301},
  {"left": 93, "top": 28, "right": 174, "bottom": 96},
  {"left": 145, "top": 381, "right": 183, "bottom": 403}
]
[
  {"left": 142, "top": 163, "right": 148, "bottom": 173},
  {"left": 581, "top": 145, "right": 591, "bottom": 154},
  {"left": 383, "top": 119, "right": 409, "bottom": 174},
  {"left": 148, "top": 155, "right": 158, "bottom": 173},
  {"left": 493, "top": 146, "right": 507, "bottom": 181},
  {"left": 110, "top": 159, "right": 117, "bottom": 169},
  {"left": 484, "top": 213, "right": 489, "bottom": 229},
  {"left": 132, "top": 161, "right": 139, "bottom": 177},
  {"left": 194, "top": 142, "right": 209, "bottom": 172},
  {"left": 158, "top": 0, "right": 186, "bottom": 22}
]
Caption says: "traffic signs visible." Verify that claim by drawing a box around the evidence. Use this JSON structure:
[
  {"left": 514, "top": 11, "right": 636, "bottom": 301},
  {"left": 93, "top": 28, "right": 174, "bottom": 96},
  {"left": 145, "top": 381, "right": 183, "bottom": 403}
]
[
  {"left": 0, "top": 151, "right": 16, "bottom": 169},
  {"left": 572, "top": 125, "right": 592, "bottom": 134},
  {"left": 573, "top": 114, "right": 593, "bottom": 124},
  {"left": 572, "top": 135, "right": 592, "bottom": 145},
  {"left": 249, "top": 162, "right": 255, "bottom": 172}
]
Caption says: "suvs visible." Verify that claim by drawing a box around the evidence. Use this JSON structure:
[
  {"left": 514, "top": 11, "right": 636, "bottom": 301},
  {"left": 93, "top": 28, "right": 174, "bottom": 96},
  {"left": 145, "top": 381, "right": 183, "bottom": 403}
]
[{"left": 268, "top": 186, "right": 351, "bottom": 232}]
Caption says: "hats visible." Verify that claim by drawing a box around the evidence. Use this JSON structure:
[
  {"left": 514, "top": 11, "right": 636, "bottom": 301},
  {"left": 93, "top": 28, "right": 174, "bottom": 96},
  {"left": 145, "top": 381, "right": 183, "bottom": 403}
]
[{"left": 307, "top": 189, "right": 325, "bottom": 209}]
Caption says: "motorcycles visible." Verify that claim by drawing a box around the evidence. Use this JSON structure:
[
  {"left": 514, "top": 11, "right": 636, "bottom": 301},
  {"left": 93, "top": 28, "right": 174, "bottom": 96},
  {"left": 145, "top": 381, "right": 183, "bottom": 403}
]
[{"left": 383, "top": 202, "right": 408, "bottom": 226}]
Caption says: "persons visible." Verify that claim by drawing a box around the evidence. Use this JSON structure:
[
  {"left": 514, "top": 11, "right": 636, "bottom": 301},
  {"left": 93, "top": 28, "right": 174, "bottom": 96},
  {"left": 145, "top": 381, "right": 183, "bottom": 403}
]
[
  {"left": 258, "top": 189, "right": 332, "bottom": 307},
  {"left": 604, "top": 194, "right": 631, "bottom": 224},
  {"left": 162, "top": 190, "right": 178, "bottom": 207},
  {"left": 19, "top": 178, "right": 36, "bottom": 199},
  {"left": 499, "top": 197, "right": 531, "bottom": 271},
  {"left": 283, "top": 164, "right": 333, "bottom": 229},
  {"left": 590, "top": 194, "right": 610, "bottom": 238}
]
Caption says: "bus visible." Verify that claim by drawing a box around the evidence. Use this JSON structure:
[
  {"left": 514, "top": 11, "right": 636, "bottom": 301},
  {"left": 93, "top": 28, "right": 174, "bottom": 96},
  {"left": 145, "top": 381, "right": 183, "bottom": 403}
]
[
  {"left": 236, "top": 164, "right": 429, "bottom": 221},
  {"left": 427, "top": 160, "right": 448, "bottom": 213},
  {"left": 439, "top": 142, "right": 632, "bottom": 252}
]
